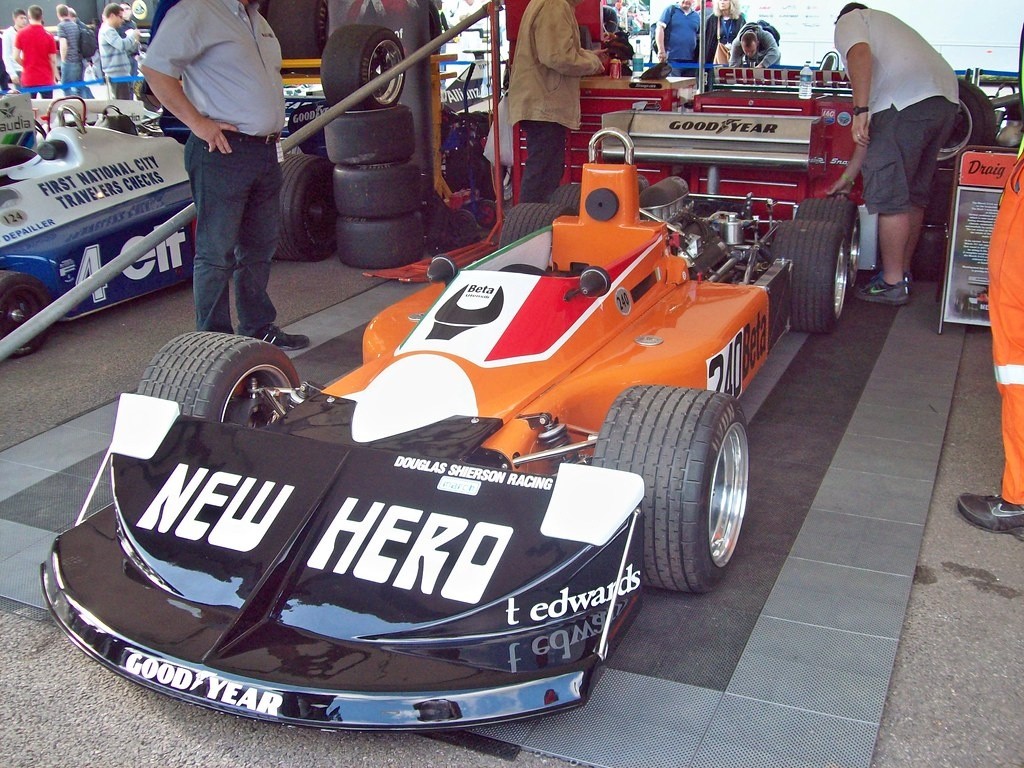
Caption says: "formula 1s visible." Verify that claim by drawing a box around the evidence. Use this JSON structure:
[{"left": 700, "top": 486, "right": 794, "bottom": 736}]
[
  {"left": 38, "top": 127, "right": 862, "bottom": 732},
  {"left": 0, "top": 97, "right": 337, "bottom": 356}
]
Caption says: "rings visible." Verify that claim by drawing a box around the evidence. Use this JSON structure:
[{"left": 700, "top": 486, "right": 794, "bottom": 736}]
[{"left": 857, "top": 134, "right": 860, "bottom": 136}]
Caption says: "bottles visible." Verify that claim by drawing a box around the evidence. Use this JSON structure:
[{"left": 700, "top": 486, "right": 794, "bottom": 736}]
[
  {"left": 798, "top": 64, "right": 813, "bottom": 100},
  {"left": 632, "top": 39, "right": 644, "bottom": 78}
]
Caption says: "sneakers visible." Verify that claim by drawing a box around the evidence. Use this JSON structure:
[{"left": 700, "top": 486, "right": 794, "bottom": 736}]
[
  {"left": 956, "top": 492, "right": 1024, "bottom": 543},
  {"left": 251, "top": 323, "right": 309, "bottom": 350},
  {"left": 853, "top": 273, "right": 914, "bottom": 307}
]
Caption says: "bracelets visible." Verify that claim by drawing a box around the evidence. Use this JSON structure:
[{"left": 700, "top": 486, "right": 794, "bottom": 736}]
[{"left": 840, "top": 172, "right": 855, "bottom": 185}]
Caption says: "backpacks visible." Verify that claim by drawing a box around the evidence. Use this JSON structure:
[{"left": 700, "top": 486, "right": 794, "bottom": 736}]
[
  {"left": 757, "top": 20, "right": 781, "bottom": 47},
  {"left": 79, "top": 28, "right": 97, "bottom": 57}
]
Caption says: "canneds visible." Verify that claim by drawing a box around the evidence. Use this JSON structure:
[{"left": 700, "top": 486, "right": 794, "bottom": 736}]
[{"left": 609, "top": 59, "right": 621, "bottom": 79}]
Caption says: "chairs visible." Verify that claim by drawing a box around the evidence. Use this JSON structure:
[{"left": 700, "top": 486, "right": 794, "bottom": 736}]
[
  {"left": 94, "top": 104, "right": 138, "bottom": 137},
  {"left": 0, "top": 144, "right": 38, "bottom": 187}
]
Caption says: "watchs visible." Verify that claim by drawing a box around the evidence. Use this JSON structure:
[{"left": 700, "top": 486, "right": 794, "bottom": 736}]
[{"left": 852, "top": 105, "right": 869, "bottom": 116}]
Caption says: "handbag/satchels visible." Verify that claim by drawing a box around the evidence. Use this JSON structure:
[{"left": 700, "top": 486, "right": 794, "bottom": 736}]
[
  {"left": 94, "top": 105, "right": 139, "bottom": 135},
  {"left": 713, "top": 43, "right": 731, "bottom": 65},
  {"left": 651, "top": 23, "right": 669, "bottom": 55},
  {"left": 483, "top": 96, "right": 513, "bottom": 166}
]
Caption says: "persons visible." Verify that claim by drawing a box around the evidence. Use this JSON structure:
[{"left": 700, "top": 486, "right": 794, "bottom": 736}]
[
  {"left": 955, "top": 151, "right": 1024, "bottom": 542},
  {"left": 139, "top": 0, "right": 311, "bottom": 352},
  {"left": 822, "top": 2, "right": 961, "bottom": 306},
  {"left": 507, "top": 0, "right": 609, "bottom": 204},
  {"left": 0, "top": 0, "right": 141, "bottom": 100},
  {"left": 655, "top": 0, "right": 781, "bottom": 91}
]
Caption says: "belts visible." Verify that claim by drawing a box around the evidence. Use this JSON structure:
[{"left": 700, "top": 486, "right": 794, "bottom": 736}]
[{"left": 222, "top": 130, "right": 278, "bottom": 145}]
